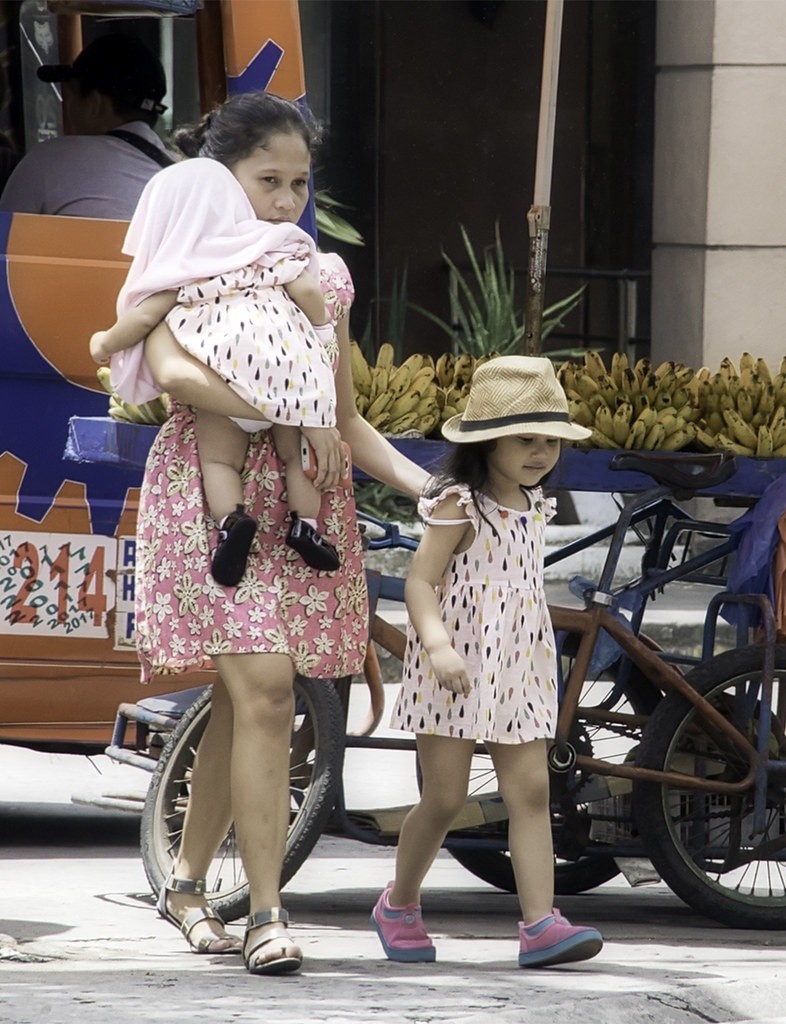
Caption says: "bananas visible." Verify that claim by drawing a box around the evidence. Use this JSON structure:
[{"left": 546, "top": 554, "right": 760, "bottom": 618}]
[
  {"left": 97, "top": 367, "right": 173, "bottom": 426},
  {"left": 552, "top": 348, "right": 786, "bottom": 459},
  {"left": 347, "top": 339, "right": 504, "bottom": 437}
]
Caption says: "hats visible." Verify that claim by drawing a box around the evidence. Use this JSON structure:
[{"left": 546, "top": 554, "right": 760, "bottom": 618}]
[
  {"left": 36, "top": 35, "right": 168, "bottom": 117},
  {"left": 442, "top": 356, "right": 593, "bottom": 444}
]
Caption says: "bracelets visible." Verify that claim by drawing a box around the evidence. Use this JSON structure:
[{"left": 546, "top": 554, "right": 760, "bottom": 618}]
[{"left": 423, "top": 475, "right": 436, "bottom": 497}]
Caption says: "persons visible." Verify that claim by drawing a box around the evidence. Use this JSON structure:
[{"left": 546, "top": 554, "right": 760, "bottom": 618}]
[
  {"left": 0, "top": 14, "right": 190, "bottom": 221},
  {"left": 137, "top": 92, "right": 454, "bottom": 975},
  {"left": 88, "top": 158, "right": 338, "bottom": 586},
  {"left": 369, "top": 353, "right": 603, "bottom": 969}
]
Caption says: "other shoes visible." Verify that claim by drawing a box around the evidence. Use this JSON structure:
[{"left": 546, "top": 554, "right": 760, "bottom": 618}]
[
  {"left": 518, "top": 907, "right": 603, "bottom": 968},
  {"left": 284, "top": 509, "right": 341, "bottom": 572},
  {"left": 209, "top": 503, "right": 257, "bottom": 587},
  {"left": 372, "top": 880, "right": 437, "bottom": 962}
]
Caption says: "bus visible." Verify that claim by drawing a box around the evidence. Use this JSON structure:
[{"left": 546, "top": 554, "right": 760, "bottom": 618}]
[{"left": 0, "top": 0, "right": 355, "bottom": 756}]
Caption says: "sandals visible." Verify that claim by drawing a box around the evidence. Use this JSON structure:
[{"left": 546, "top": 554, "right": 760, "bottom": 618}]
[
  {"left": 156, "top": 859, "right": 244, "bottom": 954},
  {"left": 242, "top": 907, "right": 303, "bottom": 972}
]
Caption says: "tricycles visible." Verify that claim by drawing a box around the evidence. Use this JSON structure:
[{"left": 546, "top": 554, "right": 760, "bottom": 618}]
[{"left": 114, "top": 451, "right": 786, "bottom": 931}]
